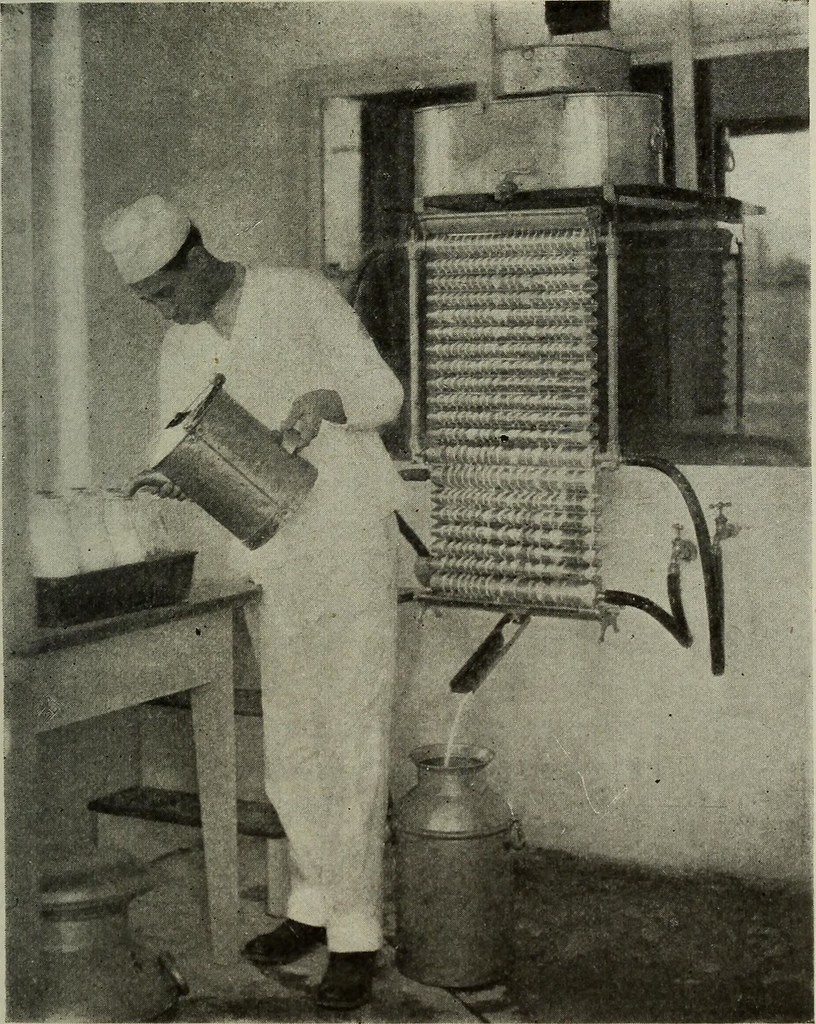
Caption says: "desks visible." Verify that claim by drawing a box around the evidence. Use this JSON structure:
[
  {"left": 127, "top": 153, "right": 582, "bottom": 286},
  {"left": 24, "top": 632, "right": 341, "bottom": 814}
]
[{"left": 29, "top": 571, "right": 264, "bottom": 968}]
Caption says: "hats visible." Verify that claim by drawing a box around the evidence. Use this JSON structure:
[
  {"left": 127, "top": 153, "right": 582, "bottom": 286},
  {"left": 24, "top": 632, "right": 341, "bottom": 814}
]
[{"left": 100, "top": 193, "right": 191, "bottom": 285}]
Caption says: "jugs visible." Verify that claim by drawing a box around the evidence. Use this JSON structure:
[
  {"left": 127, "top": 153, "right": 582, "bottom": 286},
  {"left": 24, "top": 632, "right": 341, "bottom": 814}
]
[{"left": 122, "top": 374, "right": 318, "bottom": 551}]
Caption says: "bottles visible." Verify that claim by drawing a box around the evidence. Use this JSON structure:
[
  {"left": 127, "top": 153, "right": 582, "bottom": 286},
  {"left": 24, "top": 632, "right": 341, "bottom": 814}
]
[
  {"left": 28, "top": 486, "right": 174, "bottom": 576},
  {"left": 27, "top": 841, "right": 191, "bottom": 1022},
  {"left": 391, "top": 743, "right": 527, "bottom": 988}
]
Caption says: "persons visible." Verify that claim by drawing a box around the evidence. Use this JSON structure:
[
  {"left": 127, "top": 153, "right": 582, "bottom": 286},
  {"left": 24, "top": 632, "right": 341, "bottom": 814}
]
[{"left": 100, "top": 193, "right": 405, "bottom": 1009}]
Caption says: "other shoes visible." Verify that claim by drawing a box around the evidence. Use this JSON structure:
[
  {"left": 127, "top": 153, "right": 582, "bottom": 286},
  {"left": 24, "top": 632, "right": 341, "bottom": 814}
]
[
  {"left": 315, "top": 951, "right": 373, "bottom": 1008},
  {"left": 241, "top": 918, "right": 327, "bottom": 963}
]
